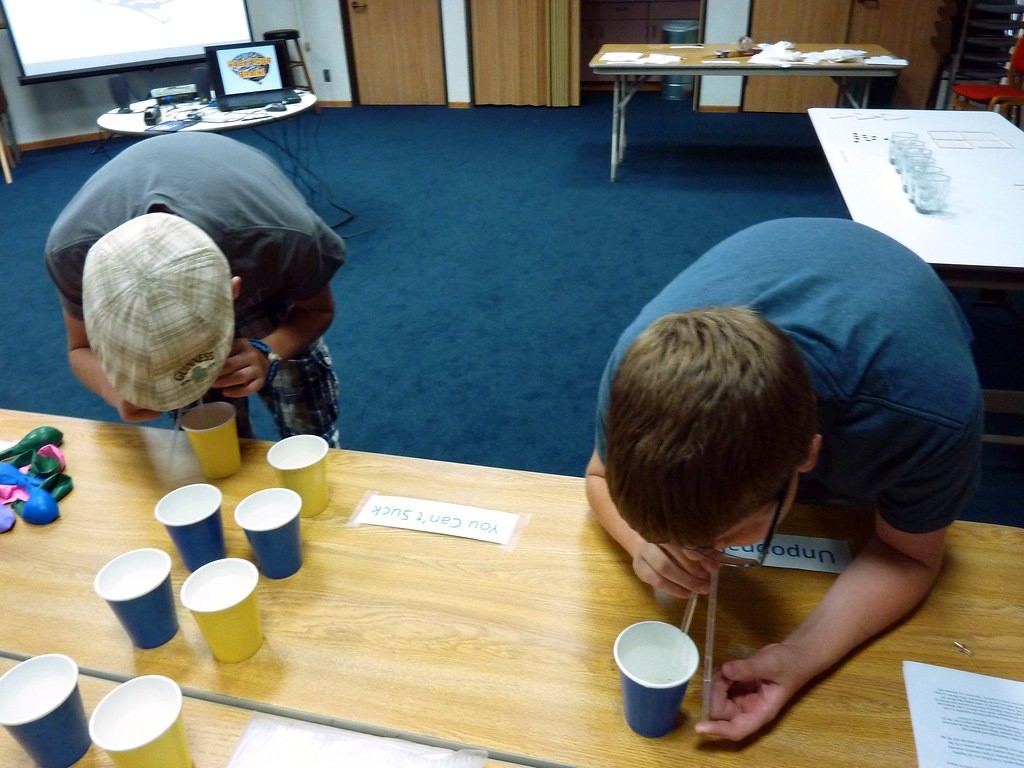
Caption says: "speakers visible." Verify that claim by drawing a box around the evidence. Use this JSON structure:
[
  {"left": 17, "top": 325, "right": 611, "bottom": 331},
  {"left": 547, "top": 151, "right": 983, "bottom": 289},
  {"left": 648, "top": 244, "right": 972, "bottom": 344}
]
[
  {"left": 106, "top": 75, "right": 134, "bottom": 113},
  {"left": 193, "top": 67, "right": 211, "bottom": 101}
]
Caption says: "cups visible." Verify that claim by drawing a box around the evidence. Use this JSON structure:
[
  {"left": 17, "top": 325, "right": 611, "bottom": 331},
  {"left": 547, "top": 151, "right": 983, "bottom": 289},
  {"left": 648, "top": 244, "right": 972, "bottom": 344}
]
[
  {"left": 87, "top": 673, "right": 193, "bottom": 768},
  {"left": 0, "top": 654, "right": 92, "bottom": 768},
  {"left": 154, "top": 483, "right": 227, "bottom": 572},
  {"left": 93, "top": 548, "right": 179, "bottom": 650},
  {"left": 234, "top": 488, "right": 303, "bottom": 579},
  {"left": 179, "top": 401, "right": 241, "bottom": 479},
  {"left": 180, "top": 557, "right": 263, "bottom": 663},
  {"left": 915, "top": 174, "right": 950, "bottom": 214},
  {"left": 613, "top": 621, "right": 699, "bottom": 738},
  {"left": 890, "top": 132, "right": 943, "bottom": 204},
  {"left": 266, "top": 435, "right": 329, "bottom": 518}
]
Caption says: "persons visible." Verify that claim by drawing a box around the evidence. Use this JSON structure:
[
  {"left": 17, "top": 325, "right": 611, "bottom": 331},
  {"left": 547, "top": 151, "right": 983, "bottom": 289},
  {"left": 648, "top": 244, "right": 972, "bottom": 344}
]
[
  {"left": 586, "top": 217, "right": 986, "bottom": 742},
  {"left": 45, "top": 130, "right": 345, "bottom": 451}
]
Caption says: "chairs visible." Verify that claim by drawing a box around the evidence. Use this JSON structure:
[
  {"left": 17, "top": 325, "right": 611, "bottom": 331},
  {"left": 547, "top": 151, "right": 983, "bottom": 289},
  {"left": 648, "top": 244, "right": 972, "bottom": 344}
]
[{"left": 950, "top": 35, "right": 1024, "bottom": 126}]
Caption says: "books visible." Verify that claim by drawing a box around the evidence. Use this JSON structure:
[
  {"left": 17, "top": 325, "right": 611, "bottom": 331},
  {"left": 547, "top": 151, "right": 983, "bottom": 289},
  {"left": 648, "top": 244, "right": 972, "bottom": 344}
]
[{"left": 144, "top": 120, "right": 201, "bottom": 133}]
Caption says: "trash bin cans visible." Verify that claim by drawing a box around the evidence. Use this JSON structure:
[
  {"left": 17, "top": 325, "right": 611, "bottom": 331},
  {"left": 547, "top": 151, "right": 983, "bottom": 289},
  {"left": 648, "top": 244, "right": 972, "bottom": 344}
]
[{"left": 661, "top": 20, "right": 700, "bottom": 101}]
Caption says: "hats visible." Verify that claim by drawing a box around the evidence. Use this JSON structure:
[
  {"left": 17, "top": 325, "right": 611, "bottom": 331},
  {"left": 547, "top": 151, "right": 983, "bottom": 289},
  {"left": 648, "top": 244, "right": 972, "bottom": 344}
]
[{"left": 84, "top": 213, "right": 236, "bottom": 411}]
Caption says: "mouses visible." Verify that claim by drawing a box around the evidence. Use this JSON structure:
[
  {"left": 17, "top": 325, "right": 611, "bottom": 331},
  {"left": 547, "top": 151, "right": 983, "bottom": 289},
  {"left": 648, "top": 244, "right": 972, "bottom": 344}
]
[{"left": 265, "top": 102, "right": 288, "bottom": 112}]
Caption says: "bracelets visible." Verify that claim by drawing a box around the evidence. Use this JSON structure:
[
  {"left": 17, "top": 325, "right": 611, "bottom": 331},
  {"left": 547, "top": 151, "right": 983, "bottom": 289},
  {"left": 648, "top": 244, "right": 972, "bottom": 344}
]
[{"left": 247, "top": 338, "right": 282, "bottom": 384}]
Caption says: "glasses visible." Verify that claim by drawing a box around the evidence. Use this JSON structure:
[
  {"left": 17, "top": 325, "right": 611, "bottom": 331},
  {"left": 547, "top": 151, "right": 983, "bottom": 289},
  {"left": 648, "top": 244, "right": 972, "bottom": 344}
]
[{"left": 685, "top": 466, "right": 795, "bottom": 569}]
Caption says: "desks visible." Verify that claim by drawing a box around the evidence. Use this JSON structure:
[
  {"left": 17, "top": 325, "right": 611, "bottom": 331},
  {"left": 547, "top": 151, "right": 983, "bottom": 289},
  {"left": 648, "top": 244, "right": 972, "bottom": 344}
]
[
  {"left": 97, "top": 89, "right": 316, "bottom": 161},
  {"left": 589, "top": 44, "right": 906, "bottom": 182},
  {"left": 805, "top": 109, "right": 1023, "bottom": 416},
  {"left": 0, "top": 405, "right": 1022, "bottom": 768}
]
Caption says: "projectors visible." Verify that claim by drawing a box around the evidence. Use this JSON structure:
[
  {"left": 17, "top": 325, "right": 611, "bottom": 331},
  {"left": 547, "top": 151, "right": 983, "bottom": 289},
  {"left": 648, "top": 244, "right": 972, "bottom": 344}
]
[{"left": 150, "top": 84, "right": 198, "bottom": 104}]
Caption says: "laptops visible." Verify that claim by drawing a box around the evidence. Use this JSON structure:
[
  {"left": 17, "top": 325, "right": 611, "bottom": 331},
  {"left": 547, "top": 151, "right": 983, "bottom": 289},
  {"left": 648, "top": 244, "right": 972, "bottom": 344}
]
[{"left": 204, "top": 38, "right": 302, "bottom": 112}]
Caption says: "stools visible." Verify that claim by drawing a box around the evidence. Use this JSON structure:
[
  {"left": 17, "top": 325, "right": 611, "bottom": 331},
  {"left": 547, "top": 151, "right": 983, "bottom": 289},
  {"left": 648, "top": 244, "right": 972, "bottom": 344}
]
[{"left": 264, "top": 29, "right": 320, "bottom": 117}]
